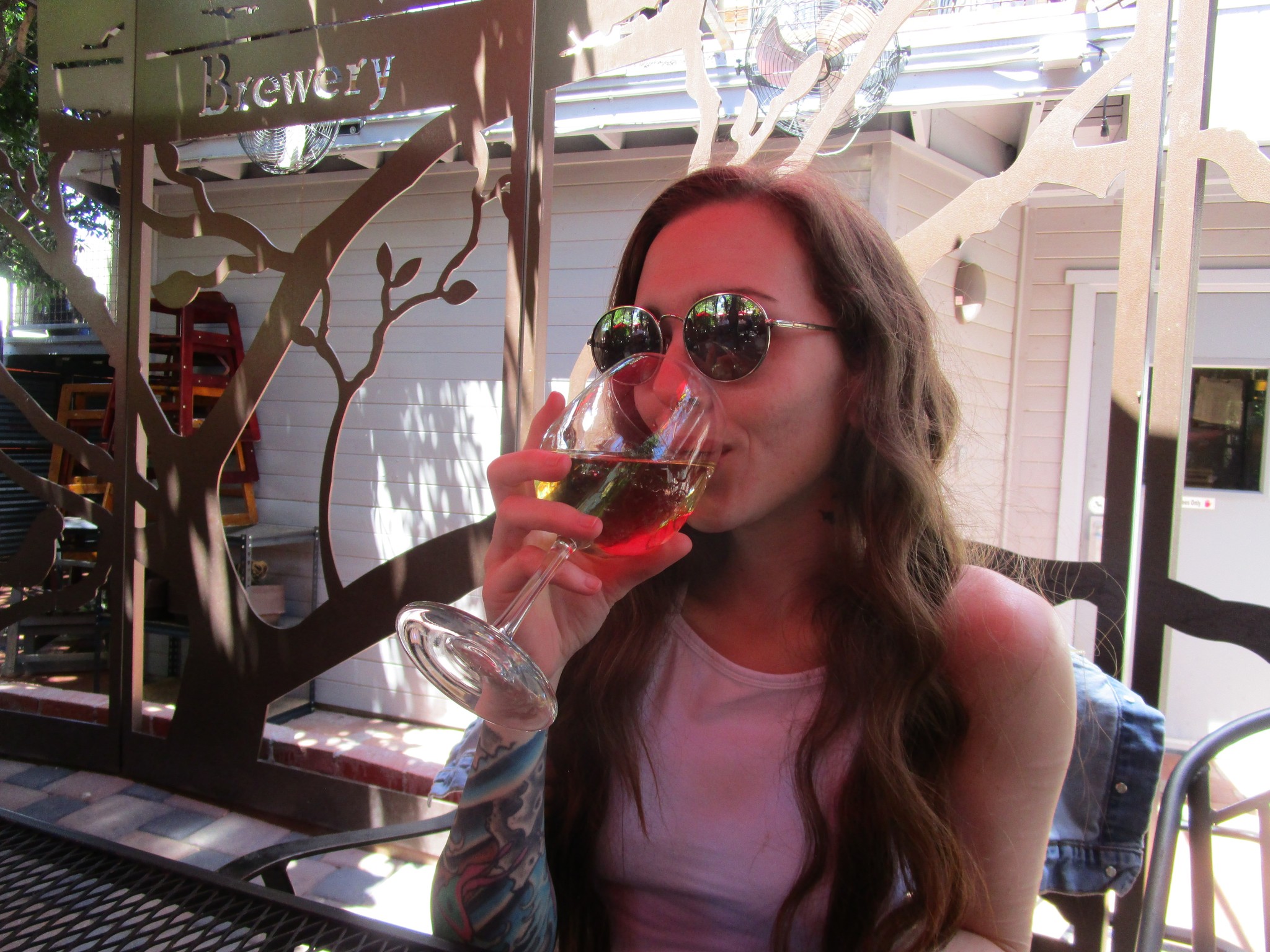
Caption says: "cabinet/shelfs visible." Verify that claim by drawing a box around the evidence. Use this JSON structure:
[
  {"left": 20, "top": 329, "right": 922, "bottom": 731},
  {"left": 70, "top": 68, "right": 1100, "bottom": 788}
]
[{"left": 225, "top": 522, "right": 319, "bottom": 724}]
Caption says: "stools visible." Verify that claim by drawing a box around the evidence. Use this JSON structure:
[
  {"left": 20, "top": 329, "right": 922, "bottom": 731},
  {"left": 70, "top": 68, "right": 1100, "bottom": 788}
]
[{"left": 50, "top": 292, "right": 261, "bottom": 526}]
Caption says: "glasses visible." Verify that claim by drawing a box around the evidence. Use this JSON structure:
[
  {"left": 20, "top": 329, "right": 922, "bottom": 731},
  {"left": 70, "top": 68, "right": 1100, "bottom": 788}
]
[{"left": 587, "top": 292, "right": 842, "bottom": 386}]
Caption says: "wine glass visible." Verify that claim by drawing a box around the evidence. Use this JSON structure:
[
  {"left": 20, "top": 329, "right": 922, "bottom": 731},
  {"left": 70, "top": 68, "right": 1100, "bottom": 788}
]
[{"left": 394, "top": 351, "right": 727, "bottom": 732}]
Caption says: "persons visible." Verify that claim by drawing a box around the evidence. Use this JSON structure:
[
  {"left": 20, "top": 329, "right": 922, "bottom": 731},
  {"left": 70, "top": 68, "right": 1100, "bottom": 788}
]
[{"left": 431, "top": 165, "right": 1078, "bottom": 952}]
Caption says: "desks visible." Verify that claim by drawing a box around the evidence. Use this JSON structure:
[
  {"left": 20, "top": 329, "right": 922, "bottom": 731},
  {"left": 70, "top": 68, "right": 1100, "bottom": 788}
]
[{"left": 0, "top": 808, "right": 458, "bottom": 952}]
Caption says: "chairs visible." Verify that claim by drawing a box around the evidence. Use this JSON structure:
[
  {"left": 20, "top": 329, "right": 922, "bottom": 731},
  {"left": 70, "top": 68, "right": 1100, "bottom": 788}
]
[{"left": 216, "top": 648, "right": 1270, "bottom": 952}]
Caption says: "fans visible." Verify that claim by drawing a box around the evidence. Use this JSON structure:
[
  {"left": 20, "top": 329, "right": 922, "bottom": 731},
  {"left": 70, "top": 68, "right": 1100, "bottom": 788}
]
[{"left": 735, "top": 0, "right": 911, "bottom": 155}]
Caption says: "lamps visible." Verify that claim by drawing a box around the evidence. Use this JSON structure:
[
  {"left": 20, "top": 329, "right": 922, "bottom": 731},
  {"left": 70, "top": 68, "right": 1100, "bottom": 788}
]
[{"left": 954, "top": 261, "right": 987, "bottom": 324}]
